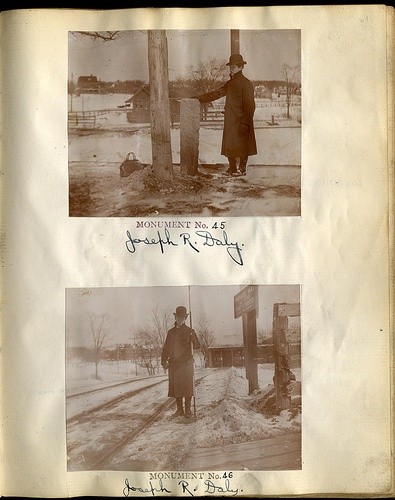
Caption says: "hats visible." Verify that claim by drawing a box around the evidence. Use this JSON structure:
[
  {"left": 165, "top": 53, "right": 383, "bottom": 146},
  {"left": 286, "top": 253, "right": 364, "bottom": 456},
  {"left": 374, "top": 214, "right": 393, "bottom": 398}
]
[
  {"left": 226, "top": 53, "right": 247, "bottom": 66},
  {"left": 173, "top": 306, "right": 190, "bottom": 316}
]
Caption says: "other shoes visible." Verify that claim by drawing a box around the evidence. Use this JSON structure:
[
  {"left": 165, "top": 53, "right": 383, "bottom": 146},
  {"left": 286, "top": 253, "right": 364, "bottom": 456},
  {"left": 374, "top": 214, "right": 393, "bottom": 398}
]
[
  {"left": 185, "top": 408, "right": 192, "bottom": 417},
  {"left": 173, "top": 409, "right": 184, "bottom": 416}
]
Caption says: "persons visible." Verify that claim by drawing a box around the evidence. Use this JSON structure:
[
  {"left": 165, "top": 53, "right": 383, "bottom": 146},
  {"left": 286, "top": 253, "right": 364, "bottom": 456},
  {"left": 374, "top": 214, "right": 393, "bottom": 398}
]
[
  {"left": 161, "top": 305, "right": 202, "bottom": 420},
  {"left": 190, "top": 54, "right": 257, "bottom": 177}
]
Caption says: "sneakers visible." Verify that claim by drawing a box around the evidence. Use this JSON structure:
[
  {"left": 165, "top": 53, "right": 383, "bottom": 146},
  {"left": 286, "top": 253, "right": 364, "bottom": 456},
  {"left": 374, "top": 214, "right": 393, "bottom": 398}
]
[
  {"left": 222, "top": 168, "right": 238, "bottom": 175},
  {"left": 233, "top": 169, "right": 247, "bottom": 176}
]
